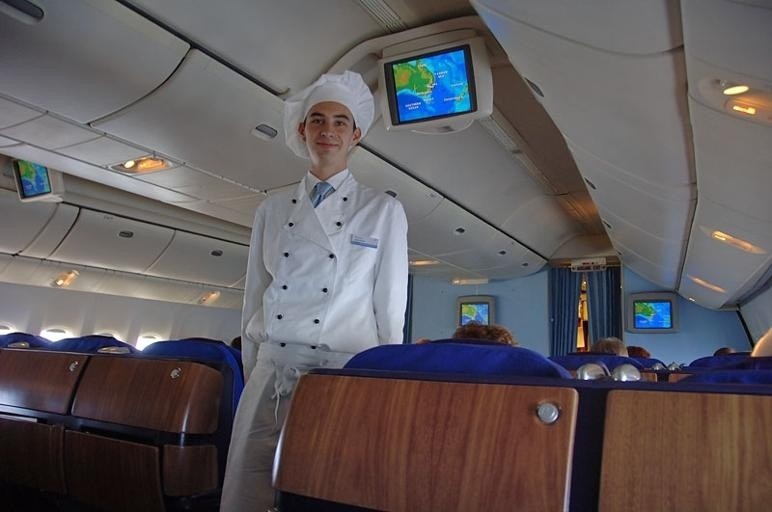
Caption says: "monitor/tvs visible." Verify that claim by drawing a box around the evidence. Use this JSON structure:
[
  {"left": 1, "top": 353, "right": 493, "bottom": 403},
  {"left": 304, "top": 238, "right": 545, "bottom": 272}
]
[
  {"left": 378, "top": 38, "right": 494, "bottom": 135},
  {"left": 11, "top": 158, "right": 65, "bottom": 202},
  {"left": 626, "top": 292, "right": 678, "bottom": 334},
  {"left": 457, "top": 297, "right": 495, "bottom": 328}
]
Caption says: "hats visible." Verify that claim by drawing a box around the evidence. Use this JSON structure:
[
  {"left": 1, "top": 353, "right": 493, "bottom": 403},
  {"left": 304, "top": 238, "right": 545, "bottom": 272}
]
[{"left": 284, "top": 71, "right": 375, "bottom": 159}]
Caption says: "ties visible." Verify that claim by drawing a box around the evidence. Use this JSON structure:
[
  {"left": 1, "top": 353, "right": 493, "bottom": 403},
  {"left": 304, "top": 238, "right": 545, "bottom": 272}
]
[{"left": 310, "top": 181, "right": 332, "bottom": 208}]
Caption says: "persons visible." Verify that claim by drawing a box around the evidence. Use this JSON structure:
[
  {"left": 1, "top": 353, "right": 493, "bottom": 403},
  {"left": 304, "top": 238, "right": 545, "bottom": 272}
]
[
  {"left": 715, "top": 347, "right": 736, "bottom": 356},
  {"left": 231, "top": 336, "right": 242, "bottom": 346},
  {"left": 219, "top": 68, "right": 410, "bottom": 510},
  {"left": 751, "top": 328, "right": 772, "bottom": 357},
  {"left": 593, "top": 337, "right": 629, "bottom": 357},
  {"left": 626, "top": 346, "right": 650, "bottom": 358},
  {"left": 415, "top": 325, "right": 513, "bottom": 345}
]
